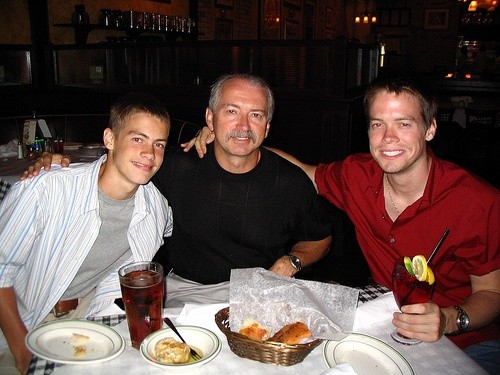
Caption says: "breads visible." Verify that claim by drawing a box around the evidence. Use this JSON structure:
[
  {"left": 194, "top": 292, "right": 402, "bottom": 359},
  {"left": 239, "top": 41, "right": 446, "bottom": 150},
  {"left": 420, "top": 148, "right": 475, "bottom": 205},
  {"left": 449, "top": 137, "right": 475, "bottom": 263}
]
[
  {"left": 155, "top": 337, "right": 191, "bottom": 364},
  {"left": 238, "top": 319, "right": 272, "bottom": 343},
  {"left": 266, "top": 322, "right": 312, "bottom": 345}
]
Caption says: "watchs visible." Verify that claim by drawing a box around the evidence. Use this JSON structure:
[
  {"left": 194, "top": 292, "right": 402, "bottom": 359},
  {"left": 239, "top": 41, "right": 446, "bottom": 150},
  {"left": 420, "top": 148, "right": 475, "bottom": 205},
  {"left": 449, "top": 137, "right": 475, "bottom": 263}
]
[
  {"left": 453, "top": 305, "right": 470, "bottom": 334},
  {"left": 285, "top": 253, "right": 302, "bottom": 272}
]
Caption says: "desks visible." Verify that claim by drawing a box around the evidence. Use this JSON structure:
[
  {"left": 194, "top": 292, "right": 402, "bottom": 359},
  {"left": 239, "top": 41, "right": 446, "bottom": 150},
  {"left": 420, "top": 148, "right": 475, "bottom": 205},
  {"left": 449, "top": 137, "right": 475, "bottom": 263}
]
[
  {"left": 25, "top": 282, "right": 489, "bottom": 375},
  {"left": 0, "top": 142, "right": 110, "bottom": 177}
]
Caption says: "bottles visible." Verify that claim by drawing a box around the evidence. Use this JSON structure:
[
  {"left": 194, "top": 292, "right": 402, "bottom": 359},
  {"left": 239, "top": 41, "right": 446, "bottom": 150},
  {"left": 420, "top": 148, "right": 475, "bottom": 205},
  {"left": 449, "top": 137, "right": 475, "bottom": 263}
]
[
  {"left": 73, "top": 4, "right": 90, "bottom": 24},
  {"left": 98, "top": 9, "right": 130, "bottom": 27},
  {"left": 31, "top": 110, "right": 42, "bottom": 139},
  {"left": 18, "top": 142, "right": 23, "bottom": 160}
]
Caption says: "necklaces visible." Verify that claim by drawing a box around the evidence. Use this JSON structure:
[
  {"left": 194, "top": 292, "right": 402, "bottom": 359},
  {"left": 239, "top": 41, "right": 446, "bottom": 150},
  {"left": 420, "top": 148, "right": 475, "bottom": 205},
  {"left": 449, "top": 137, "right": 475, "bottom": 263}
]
[{"left": 385, "top": 173, "right": 400, "bottom": 214}]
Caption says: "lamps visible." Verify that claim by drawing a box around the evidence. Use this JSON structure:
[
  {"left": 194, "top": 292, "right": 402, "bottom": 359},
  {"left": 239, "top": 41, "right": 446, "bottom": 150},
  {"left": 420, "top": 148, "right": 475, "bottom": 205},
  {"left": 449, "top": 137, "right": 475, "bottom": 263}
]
[{"left": 355, "top": 10, "right": 377, "bottom": 23}]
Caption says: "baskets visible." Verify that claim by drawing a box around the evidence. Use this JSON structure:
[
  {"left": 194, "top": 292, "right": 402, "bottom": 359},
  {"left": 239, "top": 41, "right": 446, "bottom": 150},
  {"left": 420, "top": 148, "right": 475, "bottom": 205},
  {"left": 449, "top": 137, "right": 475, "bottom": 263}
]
[{"left": 214, "top": 307, "right": 325, "bottom": 366}]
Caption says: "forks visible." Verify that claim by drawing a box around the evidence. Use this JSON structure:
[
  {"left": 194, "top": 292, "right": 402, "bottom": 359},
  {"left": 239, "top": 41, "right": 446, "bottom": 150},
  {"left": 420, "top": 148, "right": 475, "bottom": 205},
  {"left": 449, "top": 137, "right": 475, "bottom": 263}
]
[{"left": 163, "top": 317, "right": 202, "bottom": 361}]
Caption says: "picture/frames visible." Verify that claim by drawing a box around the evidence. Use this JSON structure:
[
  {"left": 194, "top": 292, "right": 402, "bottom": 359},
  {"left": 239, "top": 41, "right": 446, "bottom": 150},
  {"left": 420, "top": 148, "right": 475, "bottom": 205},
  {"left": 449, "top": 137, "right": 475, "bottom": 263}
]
[
  {"left": 422, "top": 6, "right": 452, "bottom": 31},
  {"left": 325, "top": 5, "right": 333, "bottom": 30}
]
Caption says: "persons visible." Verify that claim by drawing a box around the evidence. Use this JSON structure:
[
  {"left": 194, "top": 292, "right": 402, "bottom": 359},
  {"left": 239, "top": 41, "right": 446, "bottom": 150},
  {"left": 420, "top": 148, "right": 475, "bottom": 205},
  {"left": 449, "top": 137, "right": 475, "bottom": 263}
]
[
  {"left": 0, "top": 92, "right": 173, "bottom": 375},
  {"left": 181, "top": 72, "right": 500, "bottom": 374},
  {"left": 21, "top": 74, "right": 333, "bottom": 287}
]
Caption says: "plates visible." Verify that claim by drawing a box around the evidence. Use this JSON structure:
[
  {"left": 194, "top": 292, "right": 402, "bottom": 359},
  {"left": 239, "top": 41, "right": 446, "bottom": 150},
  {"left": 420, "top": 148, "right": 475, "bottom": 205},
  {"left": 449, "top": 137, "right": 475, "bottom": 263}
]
[
  {"left": 63, "top": 144, "right": 83, "bottom": 150},
  {"left": 140, "top": 327, "right": 222, "bottom": 370},
  {"left": 323, "top": 331, "right": 415, "bottom": 375},
  {"left": 25, "top": 319, "right": 125, "bottom": 364}
]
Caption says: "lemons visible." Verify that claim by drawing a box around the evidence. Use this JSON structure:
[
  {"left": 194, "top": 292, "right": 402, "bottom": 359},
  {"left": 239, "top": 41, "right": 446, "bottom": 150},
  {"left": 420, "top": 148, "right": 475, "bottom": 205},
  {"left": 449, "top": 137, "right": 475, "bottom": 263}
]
[{"left": 404, "top": 254, "right": 435, "bottom": 285}]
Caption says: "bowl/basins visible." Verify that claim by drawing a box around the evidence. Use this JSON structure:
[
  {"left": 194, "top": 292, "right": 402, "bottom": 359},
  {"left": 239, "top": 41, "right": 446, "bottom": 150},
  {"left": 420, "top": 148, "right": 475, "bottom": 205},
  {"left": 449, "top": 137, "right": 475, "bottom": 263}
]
[{"left": 79, "top": 144, "right": 102, "bottom": 155}]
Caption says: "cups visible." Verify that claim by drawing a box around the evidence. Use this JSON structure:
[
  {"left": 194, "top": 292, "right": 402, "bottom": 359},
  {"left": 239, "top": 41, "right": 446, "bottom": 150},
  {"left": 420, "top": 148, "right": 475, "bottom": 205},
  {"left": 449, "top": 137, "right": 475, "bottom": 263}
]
[
  {"left": 24, "top": 140, "right": 52, "bottom": 161},
  {"left": 126, "top": 11, "right": 195, "bottom": 33},
  {"left": 118, "top": 261, "right": 165, "bottom": 351},
  {"left": 54, "top": 137, "right": 64, "bottom": 154}
]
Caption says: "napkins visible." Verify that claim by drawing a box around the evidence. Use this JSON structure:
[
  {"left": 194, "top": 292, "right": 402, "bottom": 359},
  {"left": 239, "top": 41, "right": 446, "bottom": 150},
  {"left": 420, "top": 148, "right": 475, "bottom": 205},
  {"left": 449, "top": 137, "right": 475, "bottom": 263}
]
[
  {"left": 352, "top": 290, "right": 402, "bottom": 332},
  {"left": 176, "top": 301, "right": 231, "bottom": 342}
]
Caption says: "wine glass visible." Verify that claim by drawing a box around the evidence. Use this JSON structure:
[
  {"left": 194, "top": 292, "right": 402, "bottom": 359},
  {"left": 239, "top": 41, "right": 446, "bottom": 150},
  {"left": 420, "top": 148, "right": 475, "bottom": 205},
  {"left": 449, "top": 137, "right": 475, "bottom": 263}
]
[{"left": 392, "top": 255, "right": 430, "bottom": 346}]
